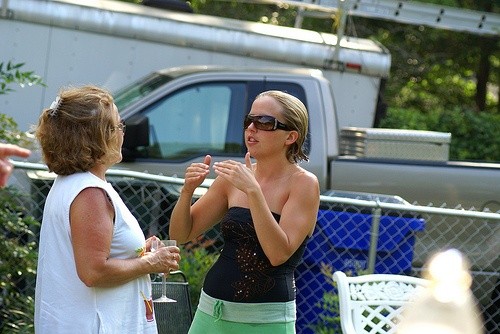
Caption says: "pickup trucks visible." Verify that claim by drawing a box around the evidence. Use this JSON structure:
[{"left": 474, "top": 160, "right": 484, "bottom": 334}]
[{"left": 31, "top": 60, "right": 500, "bottom": 282}]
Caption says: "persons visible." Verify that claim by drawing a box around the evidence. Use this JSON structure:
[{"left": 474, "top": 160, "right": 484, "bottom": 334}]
[
  {"left": 169, "top": 90, "right": 321, "bottom": 334},
  {"left": 33, "top": 86, "right": 181, "bottom": 334},
  {"left": 0, "top": 143, "right": 31, "bottom": 190}
]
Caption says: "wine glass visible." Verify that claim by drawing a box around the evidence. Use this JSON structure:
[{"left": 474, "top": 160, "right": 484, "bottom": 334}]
[{"left": 151, "top": 238, "right": 178, "bottom": 303}]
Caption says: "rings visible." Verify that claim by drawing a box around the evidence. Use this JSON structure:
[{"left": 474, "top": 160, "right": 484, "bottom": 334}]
[{"left": 173, "top": 252, "right": 175, "bottom": 260}]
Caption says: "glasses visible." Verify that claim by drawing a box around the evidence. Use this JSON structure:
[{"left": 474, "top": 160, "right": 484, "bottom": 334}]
[
  {"left": 106, "top": 120, "right": 126, "bottom": 133},
  {"left": 244, "top": 115, "right": 292, "bottom": 133}
]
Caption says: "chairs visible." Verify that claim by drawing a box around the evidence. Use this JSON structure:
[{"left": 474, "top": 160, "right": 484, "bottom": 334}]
[
  {"left": 332, "top": 271, "right": 429, "bottom": 334},
  {"left": 150, "top": 270, "right": 192, "bottom": 334}
]
